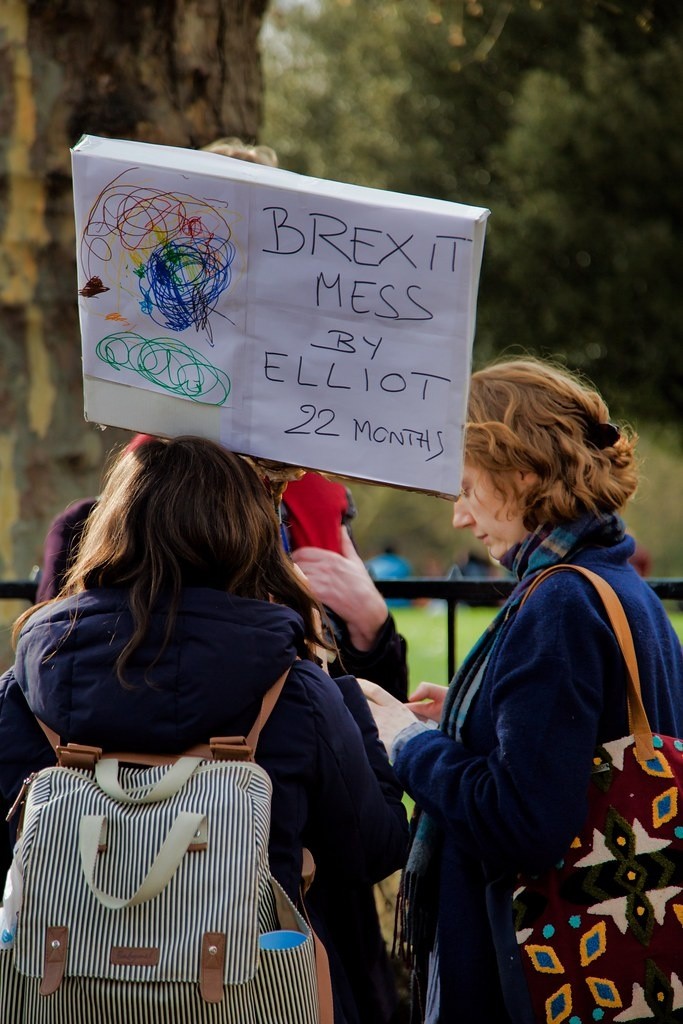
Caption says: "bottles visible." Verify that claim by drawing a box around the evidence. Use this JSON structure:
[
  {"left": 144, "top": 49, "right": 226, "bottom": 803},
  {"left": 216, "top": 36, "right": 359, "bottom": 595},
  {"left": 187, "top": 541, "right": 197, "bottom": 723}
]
[{"left": 2, "top": 924, "right": 16, "bottom": 942}]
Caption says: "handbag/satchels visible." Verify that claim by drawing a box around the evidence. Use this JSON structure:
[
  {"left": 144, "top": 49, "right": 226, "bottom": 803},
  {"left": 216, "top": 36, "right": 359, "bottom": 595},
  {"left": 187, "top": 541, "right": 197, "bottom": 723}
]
[{"left": 485, "top": 563, "right": 683, "bottom": 1024}]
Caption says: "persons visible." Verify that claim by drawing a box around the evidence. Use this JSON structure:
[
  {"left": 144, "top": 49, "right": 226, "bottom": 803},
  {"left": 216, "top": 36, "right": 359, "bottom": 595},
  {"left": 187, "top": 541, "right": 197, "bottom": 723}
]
[
  {"left": 355, "top": 354, "right": 683, "bottom": 1024},
  {"left": 0, "top": 433, "right": 405, "bottom": 1024}
]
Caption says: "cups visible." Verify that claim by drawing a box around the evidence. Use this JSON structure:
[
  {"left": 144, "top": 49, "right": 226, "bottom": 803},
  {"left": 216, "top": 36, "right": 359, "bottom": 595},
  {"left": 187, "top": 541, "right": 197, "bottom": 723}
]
[{"left": 259, "top": 930, "right": 305, "bottom": 950}]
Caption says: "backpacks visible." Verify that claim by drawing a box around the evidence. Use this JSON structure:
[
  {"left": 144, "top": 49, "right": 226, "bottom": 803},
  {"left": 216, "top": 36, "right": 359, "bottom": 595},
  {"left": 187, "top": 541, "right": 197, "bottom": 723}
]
[{"left": 0, "top": 655, "right": 334, "bottom": 1024}]
